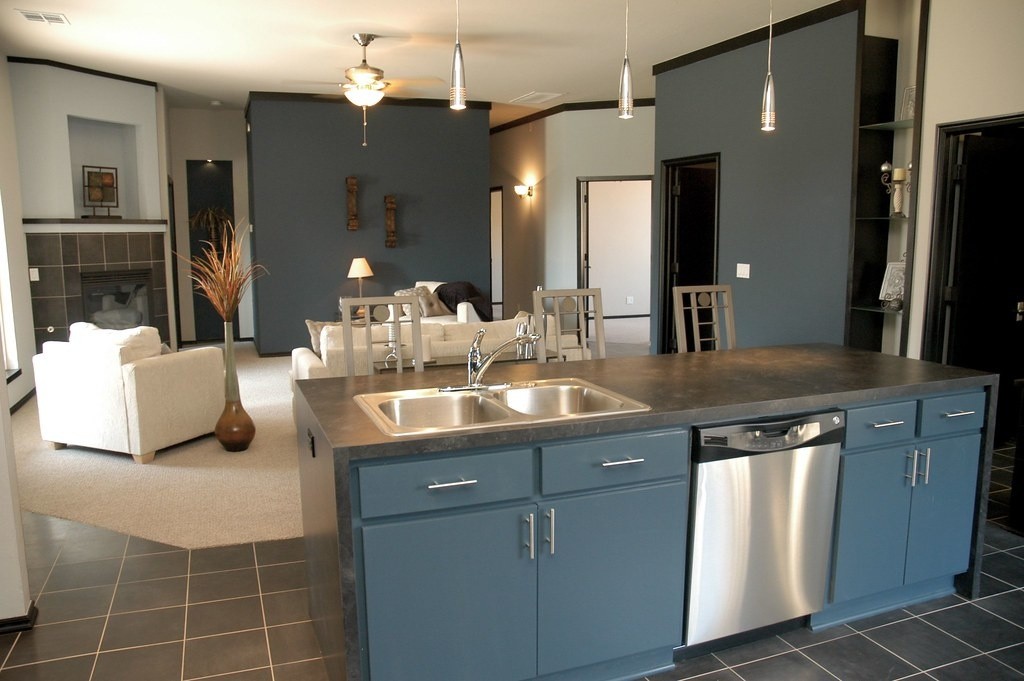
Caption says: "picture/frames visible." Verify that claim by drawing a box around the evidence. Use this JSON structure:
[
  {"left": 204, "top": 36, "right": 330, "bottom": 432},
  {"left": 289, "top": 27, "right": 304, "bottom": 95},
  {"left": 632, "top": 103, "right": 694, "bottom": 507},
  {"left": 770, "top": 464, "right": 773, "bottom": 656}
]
[
  {"left": 82, "top": 165, "right": 119, "bottom": 208},
  {"left": 878, "top": 261, "right": 905, "bottom": 301}
]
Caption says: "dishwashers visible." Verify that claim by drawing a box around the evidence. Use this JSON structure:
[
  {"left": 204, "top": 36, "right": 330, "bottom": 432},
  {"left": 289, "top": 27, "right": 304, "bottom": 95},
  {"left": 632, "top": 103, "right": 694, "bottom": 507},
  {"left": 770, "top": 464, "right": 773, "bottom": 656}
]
[{"left": 684, "top": 410, "right": 845, "bottom": 648}]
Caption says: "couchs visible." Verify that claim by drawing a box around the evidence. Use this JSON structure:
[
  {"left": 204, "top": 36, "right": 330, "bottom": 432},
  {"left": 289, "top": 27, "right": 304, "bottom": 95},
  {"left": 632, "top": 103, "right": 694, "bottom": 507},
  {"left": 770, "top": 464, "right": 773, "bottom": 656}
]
[
  {"left": 389, "top": 280, "right": 484, "bottom": 322},
  {"left": 289, "top": 314, "right": 594, "bottom": 377},
  {"left": 33, "top": 321, "right": 224, "bottom": 465}
]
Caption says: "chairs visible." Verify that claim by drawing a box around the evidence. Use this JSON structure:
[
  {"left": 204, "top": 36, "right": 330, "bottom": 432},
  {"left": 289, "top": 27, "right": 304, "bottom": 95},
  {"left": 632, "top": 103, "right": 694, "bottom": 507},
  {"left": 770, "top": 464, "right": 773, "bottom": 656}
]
[
  {"left": 340, "top": 295, "right": 424, "bottom": 373},
  {"left": 532, "top": 287, "right": 607, "bottom": 359},
  {"left": 674, "top": 284, "right": 736, "bottom": 353}
]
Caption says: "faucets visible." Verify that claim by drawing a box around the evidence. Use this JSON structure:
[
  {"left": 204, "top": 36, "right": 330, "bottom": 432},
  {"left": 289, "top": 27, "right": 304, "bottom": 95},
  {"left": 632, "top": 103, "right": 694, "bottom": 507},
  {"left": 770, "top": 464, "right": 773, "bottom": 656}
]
[{"left": 467, "top": 327, "right": 542, "bottom": 388}]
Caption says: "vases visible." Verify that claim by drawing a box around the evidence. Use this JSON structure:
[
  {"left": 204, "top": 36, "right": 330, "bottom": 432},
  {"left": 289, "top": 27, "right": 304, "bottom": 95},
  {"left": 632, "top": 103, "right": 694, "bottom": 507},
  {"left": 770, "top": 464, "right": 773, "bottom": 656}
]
[{"left": 214, "top": 322, "right": 257, "bottom": 452}]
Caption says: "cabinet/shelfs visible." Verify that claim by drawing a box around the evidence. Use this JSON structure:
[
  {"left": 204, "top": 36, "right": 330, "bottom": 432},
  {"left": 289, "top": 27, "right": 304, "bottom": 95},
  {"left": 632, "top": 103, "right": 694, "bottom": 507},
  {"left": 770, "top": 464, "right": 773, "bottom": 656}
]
[
  {"left": 351, "top": 423, "right": 694, "bottom": 681},
  {"left": 851, "top": 117, "right": 913, "bottom": 318},
  {"left": 810, "top": 390, "right": 987, "bottom": 632}
]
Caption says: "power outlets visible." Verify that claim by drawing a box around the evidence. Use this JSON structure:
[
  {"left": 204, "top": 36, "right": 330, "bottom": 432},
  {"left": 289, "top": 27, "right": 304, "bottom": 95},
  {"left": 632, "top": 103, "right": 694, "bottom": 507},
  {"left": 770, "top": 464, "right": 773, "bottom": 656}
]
[
  {"left": 306, "top": 428, "right": 315, "bottom": 458},
  {"left": 29, "top": 267, "right": 40, "bottom": 281},
  {"left": 626, "top": 296, "right": 634, "bottom": 305}
]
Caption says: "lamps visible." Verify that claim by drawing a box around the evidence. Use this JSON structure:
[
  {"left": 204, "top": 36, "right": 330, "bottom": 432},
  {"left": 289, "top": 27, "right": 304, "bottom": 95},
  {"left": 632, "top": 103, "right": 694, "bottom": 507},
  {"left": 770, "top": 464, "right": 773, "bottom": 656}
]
[
  {"left": 348, "top": 254, "right": 373, "bottom": 314},
  {"left": 513, "top": 184, "right": 533, "bottom": 199},
  {"left": 761, "top": 2, "right": 778, "bottom": 131},
  {"left": 338, "top": 34, "right": 391, "bottom": 145},
  {"left": 618, "top": 1, "right": 636, "bottom": 119},
  {"left": 449, "top": 1, "right": 466, "bottom": 112}
]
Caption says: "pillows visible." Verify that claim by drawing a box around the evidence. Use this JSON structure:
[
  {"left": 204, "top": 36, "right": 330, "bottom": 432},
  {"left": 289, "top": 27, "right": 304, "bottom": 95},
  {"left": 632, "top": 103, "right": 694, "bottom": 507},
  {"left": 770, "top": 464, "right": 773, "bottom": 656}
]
[
  {"left": 305, "top": 318, "right": 366, "bottom": 357},
  {"left": 400, "top": 285, "right": 451, "bottom": 316}
]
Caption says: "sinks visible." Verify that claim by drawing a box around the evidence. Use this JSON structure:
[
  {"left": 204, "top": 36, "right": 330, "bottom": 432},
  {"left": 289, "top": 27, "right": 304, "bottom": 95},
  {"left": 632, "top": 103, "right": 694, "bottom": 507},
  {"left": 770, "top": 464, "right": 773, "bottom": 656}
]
[
  {"left": 351, "top": 385, "right": 512, "bottom": 436},
  {"left": 490, "top": 377, "right": 652, "bottom": 420}
]
